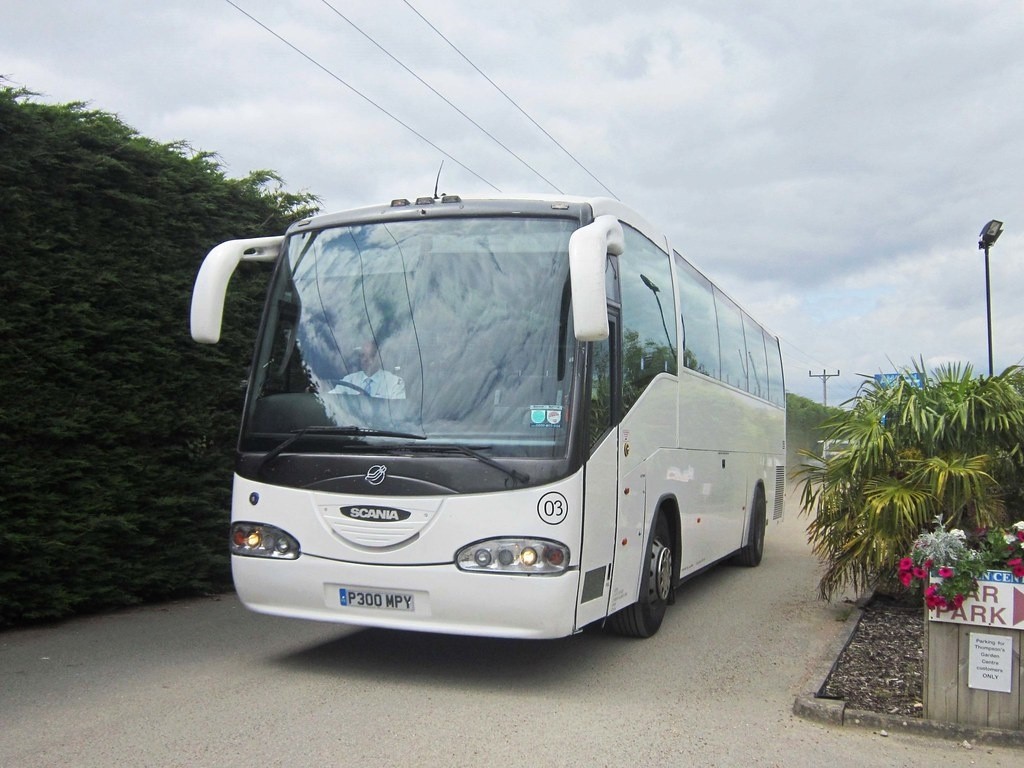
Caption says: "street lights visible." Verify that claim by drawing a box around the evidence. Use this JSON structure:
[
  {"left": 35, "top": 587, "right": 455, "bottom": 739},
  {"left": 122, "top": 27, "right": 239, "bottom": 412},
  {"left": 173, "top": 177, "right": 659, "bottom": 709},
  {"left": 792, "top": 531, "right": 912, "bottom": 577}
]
[{"left": 978, "top": 219, "right": 1004, "bottom": 379}]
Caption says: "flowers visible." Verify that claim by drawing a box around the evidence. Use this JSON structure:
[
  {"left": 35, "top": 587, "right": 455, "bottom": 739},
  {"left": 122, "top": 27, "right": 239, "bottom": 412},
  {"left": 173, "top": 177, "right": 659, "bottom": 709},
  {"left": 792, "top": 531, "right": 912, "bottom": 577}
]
[{"left": 898, "top": 510, "right": 1024, "bottom": 612}]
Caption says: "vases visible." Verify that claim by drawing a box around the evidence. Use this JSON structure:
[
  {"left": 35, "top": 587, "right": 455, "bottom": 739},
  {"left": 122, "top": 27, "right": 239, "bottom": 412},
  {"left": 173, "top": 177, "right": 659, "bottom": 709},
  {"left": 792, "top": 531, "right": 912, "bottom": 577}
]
[{"left": 922, "top": 563, "right": 1024, "bottom": 732}]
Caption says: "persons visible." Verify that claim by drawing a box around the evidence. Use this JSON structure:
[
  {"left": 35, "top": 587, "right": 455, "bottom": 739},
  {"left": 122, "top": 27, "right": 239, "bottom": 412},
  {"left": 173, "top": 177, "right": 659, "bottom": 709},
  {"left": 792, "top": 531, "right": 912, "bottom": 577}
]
[{"left": 305, "top": 341, "right": 406, "bottom": 417}]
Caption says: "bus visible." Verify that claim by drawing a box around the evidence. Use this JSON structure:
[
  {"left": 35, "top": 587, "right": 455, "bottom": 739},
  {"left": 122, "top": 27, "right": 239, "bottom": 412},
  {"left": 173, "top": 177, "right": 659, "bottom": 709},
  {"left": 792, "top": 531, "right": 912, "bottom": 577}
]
[
  {"left": 190, "top": 194, "right": 788, "bottom": 641},
  {"left": 819, "top": 438, "right": 856, "bottom": 466}
]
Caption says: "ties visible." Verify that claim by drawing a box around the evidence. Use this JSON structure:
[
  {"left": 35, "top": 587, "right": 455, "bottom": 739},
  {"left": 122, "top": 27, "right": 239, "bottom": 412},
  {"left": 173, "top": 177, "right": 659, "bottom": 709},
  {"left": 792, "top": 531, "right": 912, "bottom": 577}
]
[{"left": 363, "top": 378, "right": 373, "bottom": 394}]
